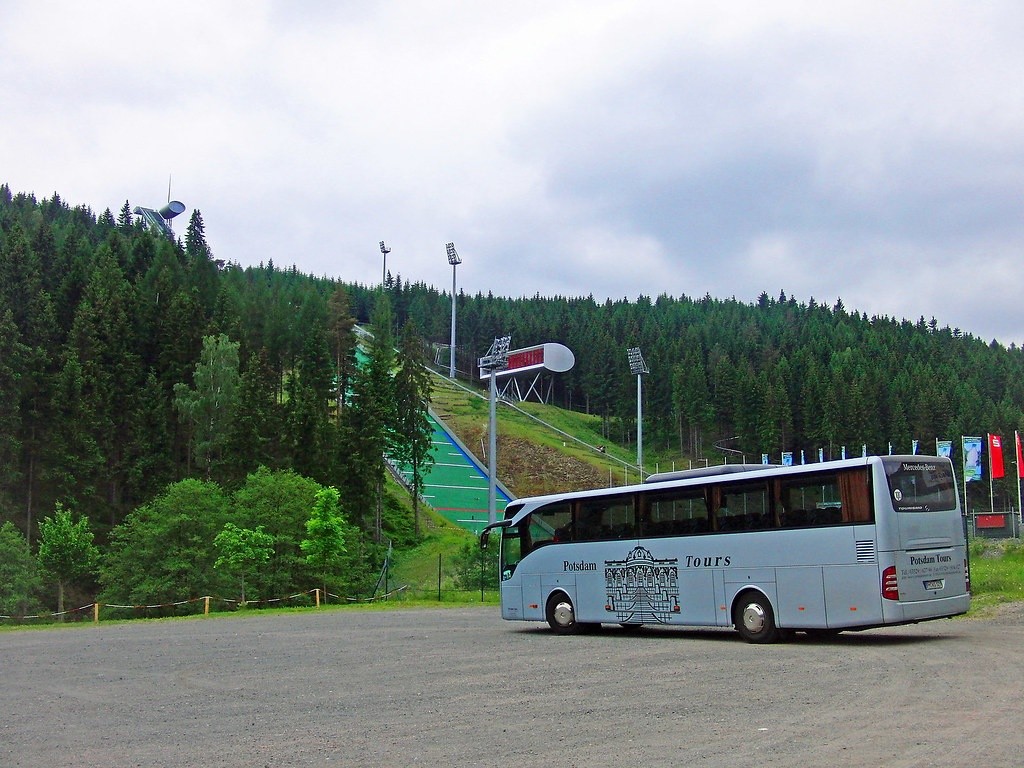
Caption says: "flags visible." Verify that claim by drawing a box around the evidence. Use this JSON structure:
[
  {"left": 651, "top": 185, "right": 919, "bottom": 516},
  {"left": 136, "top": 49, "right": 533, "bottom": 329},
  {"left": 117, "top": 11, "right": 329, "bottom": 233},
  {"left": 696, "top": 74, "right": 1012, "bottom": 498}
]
[
  {"left": 964, "top": 434, "right": 1024, "bottom": 482},
  {"left": 763, "top": 454, "right": 768, "bottom": 464},
  {"left": 783, "top": 453, "right": 792, "bottom": 466},
  {"left": 913, "top": 440, "right": 918, "bottom": 455},
  {"left": 938, "top": 441, "right": 952, "bottom": 457}
]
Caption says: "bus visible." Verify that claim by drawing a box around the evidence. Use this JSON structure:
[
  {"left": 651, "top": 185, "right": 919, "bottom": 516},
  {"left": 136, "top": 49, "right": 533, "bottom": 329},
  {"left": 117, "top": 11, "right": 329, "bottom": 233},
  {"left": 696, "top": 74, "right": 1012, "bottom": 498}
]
[{"left": 479, "top": 455, "right": 973, "bottom": 645}]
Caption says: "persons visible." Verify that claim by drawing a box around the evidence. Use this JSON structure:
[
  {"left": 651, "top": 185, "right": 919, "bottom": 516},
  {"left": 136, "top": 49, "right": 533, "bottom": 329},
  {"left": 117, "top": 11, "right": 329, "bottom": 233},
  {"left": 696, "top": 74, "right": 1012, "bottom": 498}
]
[{"left": 601, "top": 446, "right": 605, "bottom": 452}]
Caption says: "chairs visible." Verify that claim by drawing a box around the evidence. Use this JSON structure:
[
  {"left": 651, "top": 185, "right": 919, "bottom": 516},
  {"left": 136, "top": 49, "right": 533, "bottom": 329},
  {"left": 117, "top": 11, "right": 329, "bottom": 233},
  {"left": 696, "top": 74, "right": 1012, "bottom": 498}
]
[{"left": 553, "top": 507, "right": 842, "bottom": 543}]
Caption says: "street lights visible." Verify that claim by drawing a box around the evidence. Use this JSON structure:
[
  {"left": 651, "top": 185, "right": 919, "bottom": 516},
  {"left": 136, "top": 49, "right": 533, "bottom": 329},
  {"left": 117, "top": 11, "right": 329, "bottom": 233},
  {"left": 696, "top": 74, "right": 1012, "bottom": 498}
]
[
  {"left": 444, "top": 242, "right": 463, "bottom": 377},
  {"left": 485, "top": 334, "right": 512, "bottom": 535},
  {"left": 627, "top": 346, "right": 649, "bottom": 468},
  {"left": 379, "top": 240, "right": 390, "bottom": 297}
]
